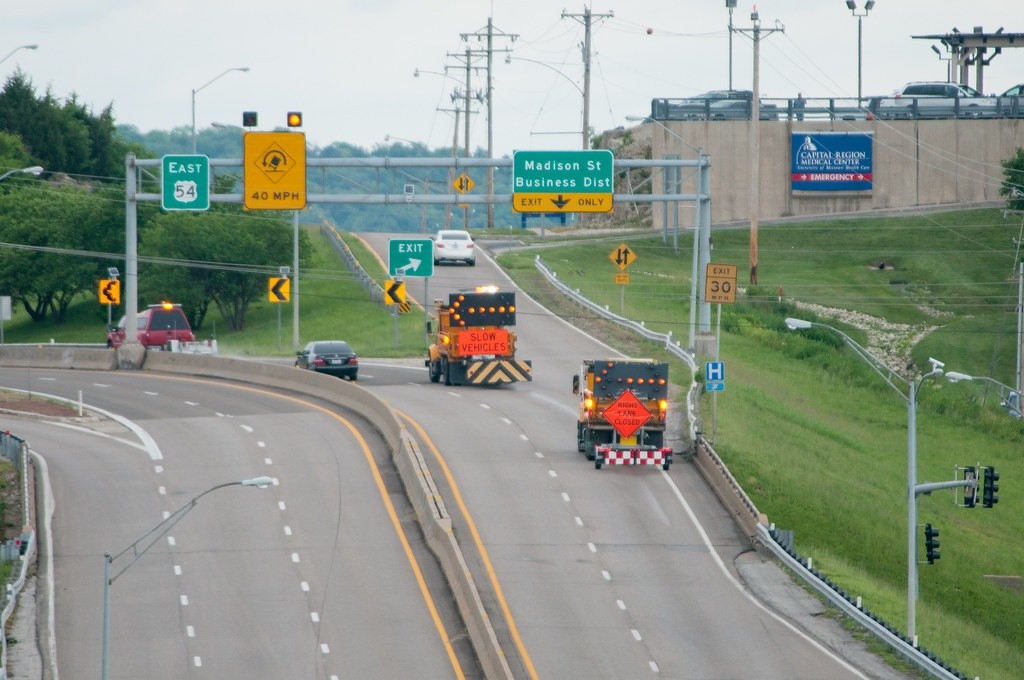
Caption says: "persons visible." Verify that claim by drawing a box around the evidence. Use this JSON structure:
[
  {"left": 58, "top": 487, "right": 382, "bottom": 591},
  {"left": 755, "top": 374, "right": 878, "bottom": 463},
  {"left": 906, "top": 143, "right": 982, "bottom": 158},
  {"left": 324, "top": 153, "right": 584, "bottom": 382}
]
[{"left": 794, "top": 92, "right": 806, "bottom": 121}]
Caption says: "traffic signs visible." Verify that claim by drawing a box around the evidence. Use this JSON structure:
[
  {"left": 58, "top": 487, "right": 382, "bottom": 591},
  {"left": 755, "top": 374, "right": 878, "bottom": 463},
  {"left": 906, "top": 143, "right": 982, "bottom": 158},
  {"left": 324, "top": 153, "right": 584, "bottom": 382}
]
[
  {"left": 387, "top": 238, "right": 434, "bottom": 279},
  {"left": 511, "top": 149, "right": 615, "bottom": 215}
]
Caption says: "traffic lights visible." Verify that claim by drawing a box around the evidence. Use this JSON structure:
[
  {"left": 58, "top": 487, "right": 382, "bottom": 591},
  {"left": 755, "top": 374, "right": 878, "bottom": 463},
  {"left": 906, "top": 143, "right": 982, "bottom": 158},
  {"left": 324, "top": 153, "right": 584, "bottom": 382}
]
[
  {"left": 982, "top": 466, "right": 1001, "bottom": 509},
  {"left": 963, "top": 465, "right": 981, "bottom": 505},
  {"left": 286, "top": 111, "right": 304, "bottom": 128},
  {"left": 923, "top": 522, "right": 941, "bottom": 564},
  {"left": 242, "top": 110, "right": 259, "bottom": 128}
]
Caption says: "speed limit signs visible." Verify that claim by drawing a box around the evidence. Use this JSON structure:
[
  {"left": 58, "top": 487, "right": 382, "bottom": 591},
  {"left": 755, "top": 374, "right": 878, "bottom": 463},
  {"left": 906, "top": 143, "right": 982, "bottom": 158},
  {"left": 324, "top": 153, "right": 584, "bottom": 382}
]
[
  {"left": 705, "top": 262, "right": 738, "bottom": 305},
  {"left": 160, "top": 154, "right": 211, "bottom": 212}
]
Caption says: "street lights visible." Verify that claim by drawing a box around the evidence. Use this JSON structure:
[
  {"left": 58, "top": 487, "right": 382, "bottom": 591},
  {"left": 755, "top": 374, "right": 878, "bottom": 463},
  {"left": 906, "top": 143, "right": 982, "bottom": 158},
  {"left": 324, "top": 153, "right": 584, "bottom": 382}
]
[
  {"left": 785, "top": 315, "right": 946, "bottom": 649},
  {"left": 502, "top": 55, "right": 591, "bottom": 226},
  {"left": 846, "top": 0, "right": 877, "bottom": 107},
  {"left": 412, "top": 66, "right": 498, "bottom": 229},
  {"left": 189, "top": 65, "right": 251, "bottom": 155},
  {"left": 623, "top": 114, "right": 703, "bottom": 357},
  {"left": 726, "top": 0, "right": 737, "bottom": 92},
  {"left": 931, "top": 45, "right": 953, "bottom": 82}
]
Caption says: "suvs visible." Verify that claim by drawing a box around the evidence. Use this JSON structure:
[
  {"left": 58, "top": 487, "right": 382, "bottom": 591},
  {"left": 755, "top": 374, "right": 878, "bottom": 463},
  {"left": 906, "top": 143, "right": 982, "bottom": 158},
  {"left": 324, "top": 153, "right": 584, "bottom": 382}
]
[{"left": 105, "top": 301, "right": 195, "bottom": 351}]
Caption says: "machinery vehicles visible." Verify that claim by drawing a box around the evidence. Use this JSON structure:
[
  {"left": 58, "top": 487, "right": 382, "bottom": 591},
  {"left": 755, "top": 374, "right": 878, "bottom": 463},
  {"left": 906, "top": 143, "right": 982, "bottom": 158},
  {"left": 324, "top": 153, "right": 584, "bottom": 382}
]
[
  {"left": 424, "top": 284, "right": 533, "bottom": 388},
  {"left": 572, "top": 356, "right": 673, "bottom": 470}
]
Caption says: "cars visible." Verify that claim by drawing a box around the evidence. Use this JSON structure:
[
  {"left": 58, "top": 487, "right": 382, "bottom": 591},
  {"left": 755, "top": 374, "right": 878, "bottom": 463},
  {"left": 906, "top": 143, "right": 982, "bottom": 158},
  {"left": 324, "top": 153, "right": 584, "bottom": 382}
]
[
  {"left": 671, "top": 87, "right": 779, "bottom": 120},
  {"left": 864, "top": 80, "right": 1024, "bottom": 120},
  {"left": 295, "top": 341, "right": 359, "bottom": 383},
  {"left": 428, "top": 229, "right": 478, "bottom": 266}
]
